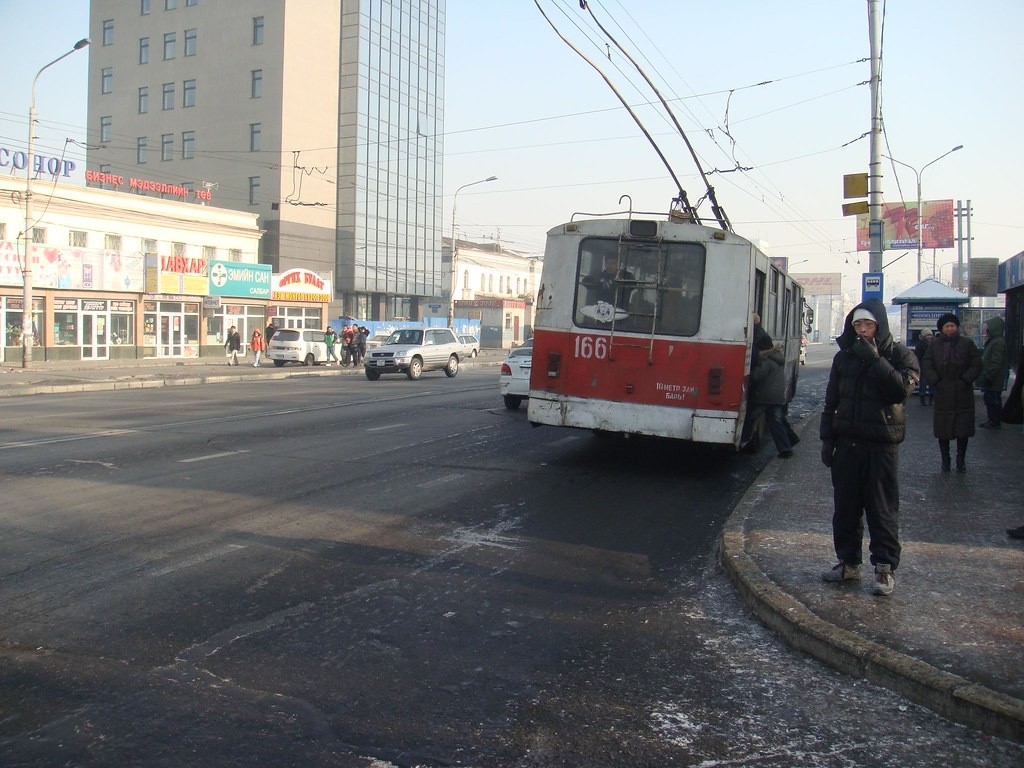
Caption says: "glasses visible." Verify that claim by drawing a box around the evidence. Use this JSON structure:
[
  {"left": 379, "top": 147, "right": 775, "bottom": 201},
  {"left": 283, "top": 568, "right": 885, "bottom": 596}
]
[{"left": 853, "top": 321, "right": 877, "bottom": 330}]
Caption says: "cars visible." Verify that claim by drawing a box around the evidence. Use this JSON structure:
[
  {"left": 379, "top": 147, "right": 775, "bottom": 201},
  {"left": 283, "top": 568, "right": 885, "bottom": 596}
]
[
  {"left": 365, "top": 334, "right": 390, "bottom": 354},
  {"left": 499, "top": 338, "right": 535, "bottom": 409}
]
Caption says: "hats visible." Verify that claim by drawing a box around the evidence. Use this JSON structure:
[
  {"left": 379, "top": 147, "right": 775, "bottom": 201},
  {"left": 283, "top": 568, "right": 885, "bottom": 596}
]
[
  {"left": 758, "top": 335, "right": 773, "bottom": 351},
  {"left": 937, "top": 314, "right": 960, "bottom": 330},
  {"left": 852, "top": 309, "right": 877, "bottom": 323},
  {"left": 920, "top": 327, "right": 933, "bottom": 338}
]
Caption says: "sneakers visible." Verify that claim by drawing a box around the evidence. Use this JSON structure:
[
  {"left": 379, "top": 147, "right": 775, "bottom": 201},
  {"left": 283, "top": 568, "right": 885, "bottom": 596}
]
[
  {"left": 872, "top": 563, "right": 896, "bottom": 596},
  {"left": 822, "top": 560, "right": 864, "bottom": 582}
]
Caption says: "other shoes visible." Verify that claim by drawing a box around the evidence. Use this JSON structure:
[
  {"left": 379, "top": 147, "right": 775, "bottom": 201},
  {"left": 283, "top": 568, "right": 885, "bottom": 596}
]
[
  {"left": 778, "top": 451, "right": 792, "bottom": 458},
  {"left": 789, "top": 436, "right": 800, "bottom": 447},
  {"left": 980, "top": 419, "right": 1002, "bottom": 428},
  {"left": 1006, "top": 525, "right": 1024, "bottom": 538},
  {"left": 942, "top": 458, "right": 951, "bottom": 471},
  {"left": 956, "top": 459, "right": 966, "bottom": 472}
]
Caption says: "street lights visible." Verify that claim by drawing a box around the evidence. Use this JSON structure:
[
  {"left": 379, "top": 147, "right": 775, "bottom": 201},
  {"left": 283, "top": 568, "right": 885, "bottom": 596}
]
[
  {"left": 447, "top": 176, "right": 500, "bottom": 329},
  {"left": 881, "top": 144, "right": 963, "bottom": 283},
  {"left": 20, "top": 38, "right": 92, "bottom": 371}
]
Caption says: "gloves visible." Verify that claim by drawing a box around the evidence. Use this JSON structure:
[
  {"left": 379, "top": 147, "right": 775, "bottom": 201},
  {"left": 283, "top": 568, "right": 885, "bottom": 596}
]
[
  {"left": 852, "top": 334, "right": 879, "bottom": 363},
  {"left": 821, "top": 441, "right": 835, "bottom": 469}
]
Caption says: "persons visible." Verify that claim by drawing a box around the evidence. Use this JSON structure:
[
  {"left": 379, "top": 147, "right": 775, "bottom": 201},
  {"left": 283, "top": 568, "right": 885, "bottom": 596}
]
[
  {"left": 819, "top": 298, "right": 920, "bottom": 596},
  {"left": 248, "top": 328, "right": 265, "bottom": 368},
  {"left": 582, "top": 252, "right": 701, "bottom": 334},
  {"left": 266, "top": 323, "right": 274, "bottom": 346},
  {"left": 915, "top": 327, "right": 936, "bottom": 407},
  {"left": 921, "top": 314, "right": 982, "bottom": 473},
  {"left": 975, "top": 316, "right": 1008, "bottom": 429},
  {"left": 526, "top": 328, "right": 533, "bottom": 339},
  {"left": 225, "top": 326, "right": 240, "bottom": 367},
  {"left": 324, "top": 324, "right": 370, "bottom": 368},
  {"left": 742, "top": 312, "right": 801, "bottom": 458}
]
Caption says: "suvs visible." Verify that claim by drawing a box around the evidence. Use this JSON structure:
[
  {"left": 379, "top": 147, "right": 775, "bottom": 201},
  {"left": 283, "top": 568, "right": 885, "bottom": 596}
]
[
  {"left": 364, "top": 327, "right": 464, "bottom": 381},
  {"left": 456, "top": 333, "right": 480, "bottom": 360}
]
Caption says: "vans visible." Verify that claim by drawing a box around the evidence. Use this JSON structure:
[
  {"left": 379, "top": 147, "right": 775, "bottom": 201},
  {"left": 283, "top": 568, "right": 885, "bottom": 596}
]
[{"left": 265, "top": 328, "right": 345, "bottom": 368}]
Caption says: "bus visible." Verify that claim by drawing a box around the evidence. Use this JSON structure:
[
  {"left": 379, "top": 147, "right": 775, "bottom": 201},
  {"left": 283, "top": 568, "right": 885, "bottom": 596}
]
[{"left": 528, "top": 217, "right": 815, "bottom": 460}]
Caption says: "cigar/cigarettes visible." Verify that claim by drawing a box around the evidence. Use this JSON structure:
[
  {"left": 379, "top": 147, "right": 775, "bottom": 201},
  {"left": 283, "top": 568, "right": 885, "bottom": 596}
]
[{"left": 858, "top": 337, "right": 860, "bottom": 340}]
